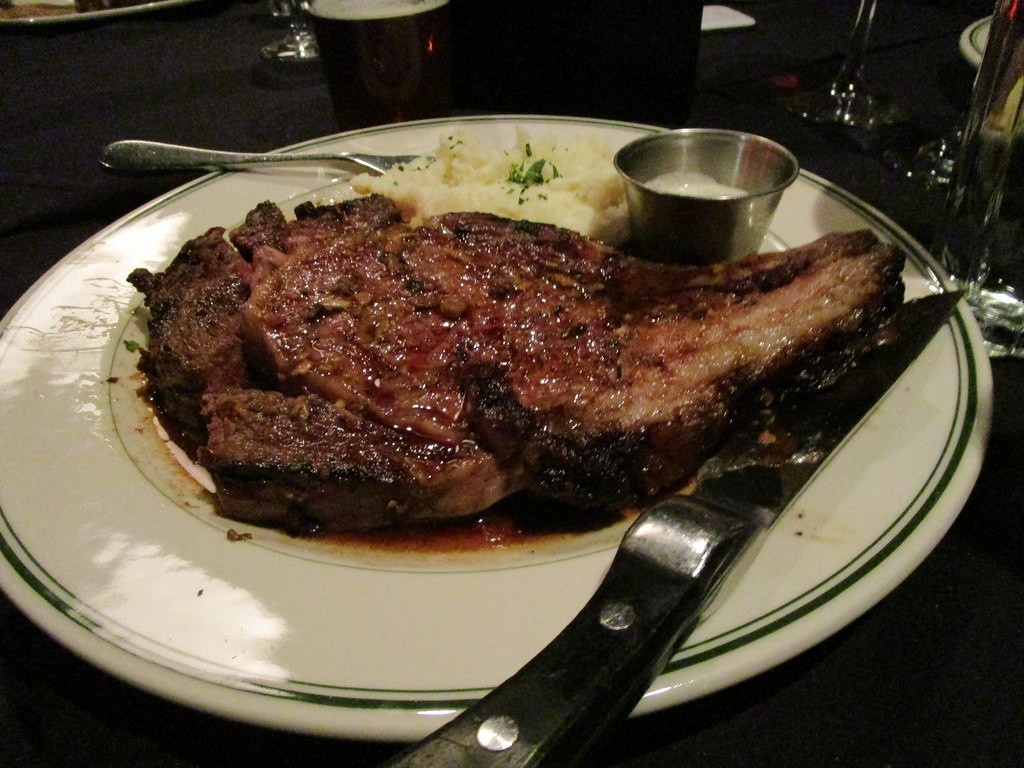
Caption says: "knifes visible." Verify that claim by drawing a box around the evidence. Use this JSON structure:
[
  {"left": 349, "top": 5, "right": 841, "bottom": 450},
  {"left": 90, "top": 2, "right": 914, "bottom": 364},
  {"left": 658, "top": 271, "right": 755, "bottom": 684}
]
[{"left": 389, "top": 290, "right": 968, "bottom": 768}]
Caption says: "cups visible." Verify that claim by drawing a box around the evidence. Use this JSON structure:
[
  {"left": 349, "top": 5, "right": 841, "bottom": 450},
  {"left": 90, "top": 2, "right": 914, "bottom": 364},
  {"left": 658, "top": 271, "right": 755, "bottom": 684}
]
[
  {"left": 929, "top": 1, "right": 1023, "bottom": 359},
  {"left": 613, "top": 126, "right": 799, "bottom": 265}
]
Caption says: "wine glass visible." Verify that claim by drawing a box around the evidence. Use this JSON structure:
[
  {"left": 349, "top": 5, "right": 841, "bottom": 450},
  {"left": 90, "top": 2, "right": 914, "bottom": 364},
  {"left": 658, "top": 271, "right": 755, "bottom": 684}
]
[{"left": 785, "top": 0, "right": 923, "bottom": 132}]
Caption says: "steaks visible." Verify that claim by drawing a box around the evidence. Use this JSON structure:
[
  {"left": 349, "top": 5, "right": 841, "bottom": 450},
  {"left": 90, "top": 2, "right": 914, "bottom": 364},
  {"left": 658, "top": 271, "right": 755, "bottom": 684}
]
[{"left": 122, "top": 194, "right": 907, "bottom": 536}]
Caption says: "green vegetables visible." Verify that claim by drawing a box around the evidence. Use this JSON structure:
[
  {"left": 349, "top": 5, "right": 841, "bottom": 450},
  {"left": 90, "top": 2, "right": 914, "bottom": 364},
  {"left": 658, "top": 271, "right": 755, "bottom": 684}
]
[{"left": 510, "top": 141, "right": 559, "bottom": 203}]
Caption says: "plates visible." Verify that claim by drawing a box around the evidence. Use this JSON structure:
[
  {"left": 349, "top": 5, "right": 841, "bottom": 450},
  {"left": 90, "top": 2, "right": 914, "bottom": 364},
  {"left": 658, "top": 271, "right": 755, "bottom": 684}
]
[{"left": 1, "top": 115, "right": 994, "bottom": 743}]
